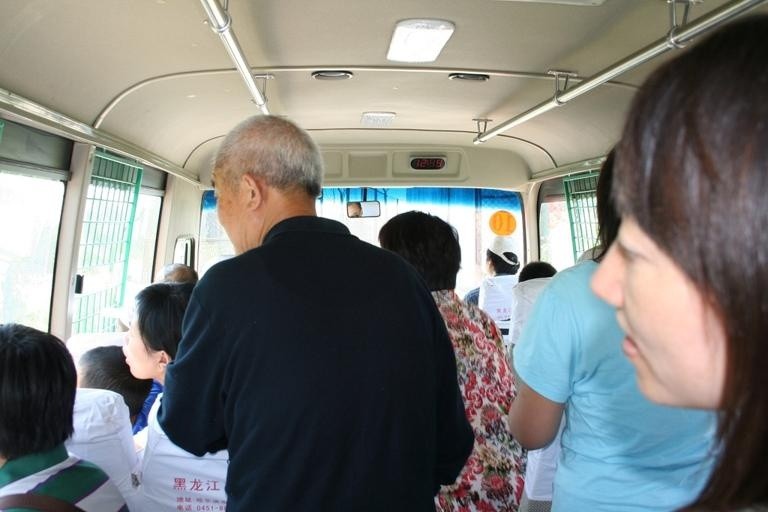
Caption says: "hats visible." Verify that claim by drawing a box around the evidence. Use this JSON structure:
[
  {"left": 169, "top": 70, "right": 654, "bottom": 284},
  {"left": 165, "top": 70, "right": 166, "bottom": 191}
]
[{"left": 488, "top": 235, "right": 520, "bottom": 267}]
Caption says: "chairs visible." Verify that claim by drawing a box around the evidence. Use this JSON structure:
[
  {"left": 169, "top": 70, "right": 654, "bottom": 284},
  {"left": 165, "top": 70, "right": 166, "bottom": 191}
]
[
  {"left": 137, "top": 394, "right": 228, "bottom": 511},
  {"left": 477, "top": 274, "right": 518, "bottom": 322},
  {"left": 518, "top": 410, "right": 561, "bottom": 512},
  {"left": 506, "top": 278, "right": 552, "bottom": 359},
  {"left": 62, "top": 386, "right": 139, "bottom": 511}
]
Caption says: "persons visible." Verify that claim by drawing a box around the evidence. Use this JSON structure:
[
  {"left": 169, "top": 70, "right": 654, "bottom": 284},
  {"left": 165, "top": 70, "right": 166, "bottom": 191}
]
[
  {"left": 76, "top": 263, "right": 199, "bottom": 435},
  {"left": 1, "top": 323, "right": 128, "bottom": 511},
  {"left": 508, "top": 146, "right": 729, "bottom": 510},
  {"left": 591, "top": 12, "right": 767, "bottom": 511},
  {"left": 379, "top": 211, "right": 528, "bottom": 511},
  {"left": 157, "top": 114, "right": 476, "bottom": 510},
  {"left": 463, "top": 244, "right": 557, "bottom": 306}
]
